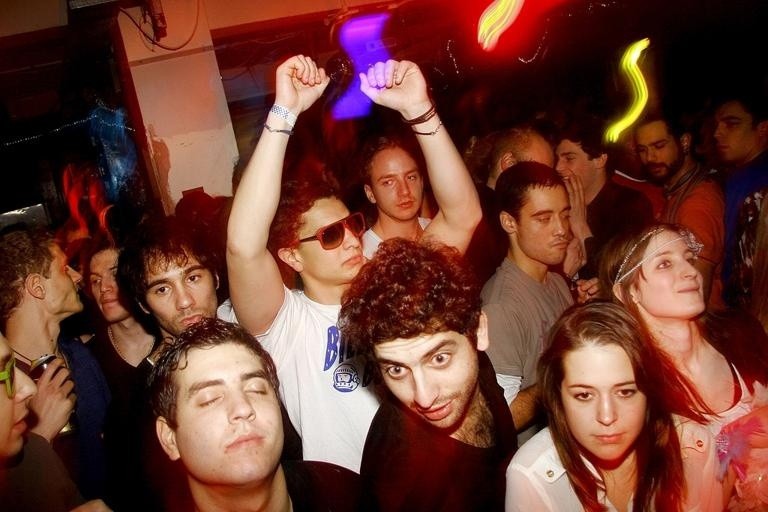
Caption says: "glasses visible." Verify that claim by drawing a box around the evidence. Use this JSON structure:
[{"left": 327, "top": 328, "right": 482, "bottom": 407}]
[
  {"left": 290, "top": 212, "right": 366, "bottom": 250},
  {"left": 0, "top": 359, "right": 15, "bottom": 398}
]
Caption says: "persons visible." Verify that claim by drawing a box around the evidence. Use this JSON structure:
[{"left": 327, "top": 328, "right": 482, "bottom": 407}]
[
  {"left": 0, "top": 98, "right": 767, "bottom": 511},
  {"left": 222, "top": 53, "right": 485, "bottom": 475}
]
